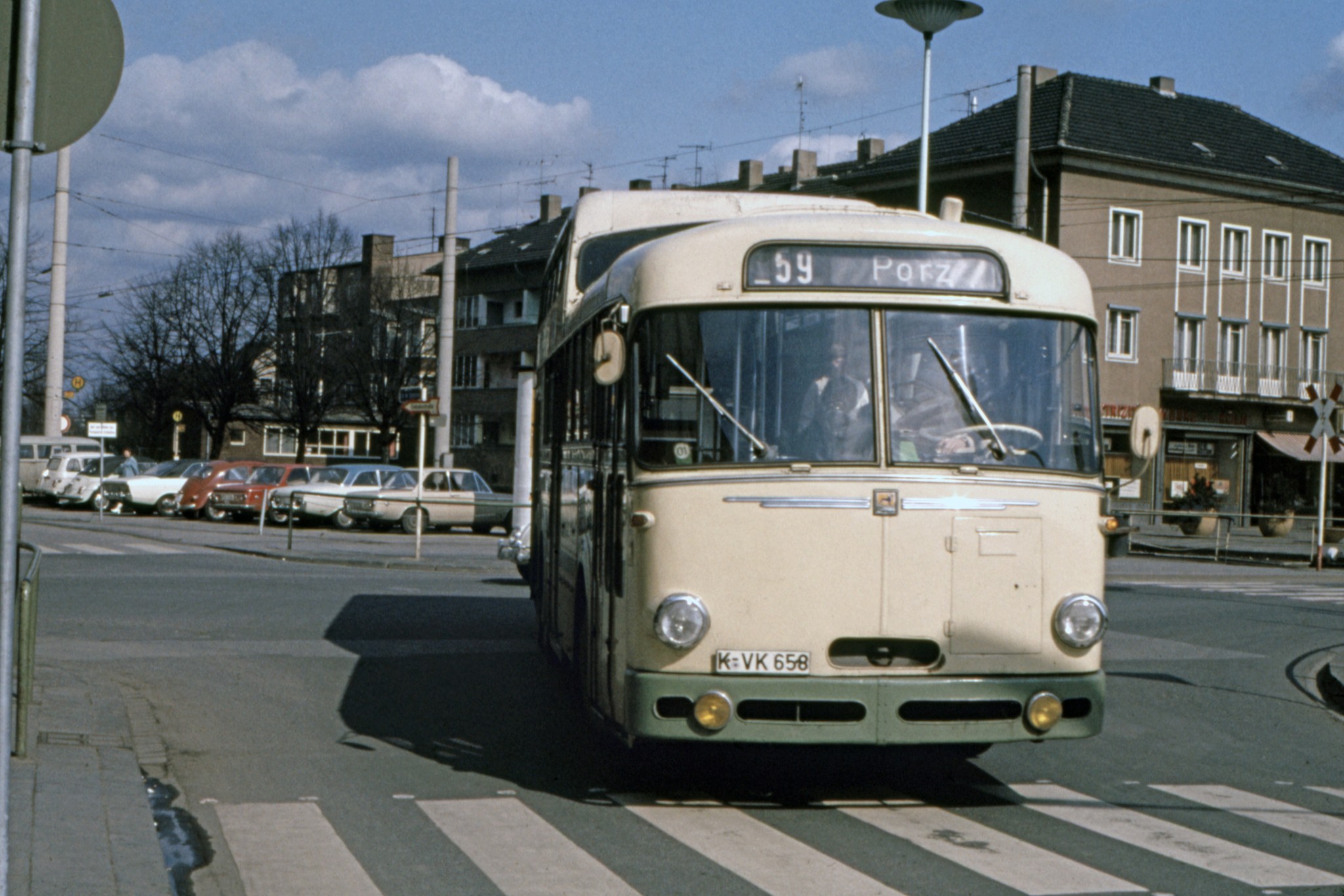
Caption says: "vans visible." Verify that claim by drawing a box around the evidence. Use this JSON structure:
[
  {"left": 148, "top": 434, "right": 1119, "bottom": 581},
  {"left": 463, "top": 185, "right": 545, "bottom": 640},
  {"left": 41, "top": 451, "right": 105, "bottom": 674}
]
[{"left": 18, "top": 435, "right": 102, "bottom": 497}]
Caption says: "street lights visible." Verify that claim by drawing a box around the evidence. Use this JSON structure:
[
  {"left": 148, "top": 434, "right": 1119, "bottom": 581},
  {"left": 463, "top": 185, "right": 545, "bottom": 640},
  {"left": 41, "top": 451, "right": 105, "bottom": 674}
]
[
  {"left": 416, "top": 369, "right": 435, "bottom": 561},
  {"left": 875, "top": 0, "right": 984, "bottom": 214}
]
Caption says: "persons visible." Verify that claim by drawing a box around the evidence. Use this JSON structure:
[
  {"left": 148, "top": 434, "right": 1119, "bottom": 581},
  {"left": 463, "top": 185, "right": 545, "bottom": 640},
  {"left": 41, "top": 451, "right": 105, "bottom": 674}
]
[
  {"left": 111, "top": 449, "right": 139, "bottom": 514},
  {"left": 797, "top": 344, "right": 869, "bottom": 432},
  {"left": 853, "top": 352, "right": 981, "bottom": 461}
]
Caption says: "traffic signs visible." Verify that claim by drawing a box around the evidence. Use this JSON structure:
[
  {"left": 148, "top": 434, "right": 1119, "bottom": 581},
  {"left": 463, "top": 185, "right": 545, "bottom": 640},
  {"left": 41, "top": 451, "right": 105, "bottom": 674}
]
[{"left": 402, "top": 398, "right": 440, "bottom": 415}]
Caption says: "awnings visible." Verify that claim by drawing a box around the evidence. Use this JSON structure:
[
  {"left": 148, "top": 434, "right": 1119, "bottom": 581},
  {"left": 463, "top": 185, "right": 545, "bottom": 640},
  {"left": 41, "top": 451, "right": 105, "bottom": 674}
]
[{"left": 1257, "top": 432, "right": 1344, "bottom": 463}]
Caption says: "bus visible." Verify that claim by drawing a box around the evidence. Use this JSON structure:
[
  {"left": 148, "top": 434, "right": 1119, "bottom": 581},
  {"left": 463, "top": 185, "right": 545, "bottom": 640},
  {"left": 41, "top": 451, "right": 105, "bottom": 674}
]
[{"left": 528, "top": 187, "right": 1160, "bottom": 759}]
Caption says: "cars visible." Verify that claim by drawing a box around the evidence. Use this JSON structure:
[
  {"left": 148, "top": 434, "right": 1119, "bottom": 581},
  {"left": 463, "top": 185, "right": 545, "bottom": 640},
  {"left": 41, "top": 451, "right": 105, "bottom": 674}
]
[
  {"left": 36, "top": 451, "right": 214, "bottom": 515},
  {"left": 174, "top": 460, "right": 414, "bottom": 530},
  {"left": 342, "top": 468, "right": 515, "bottom": 536}
]
